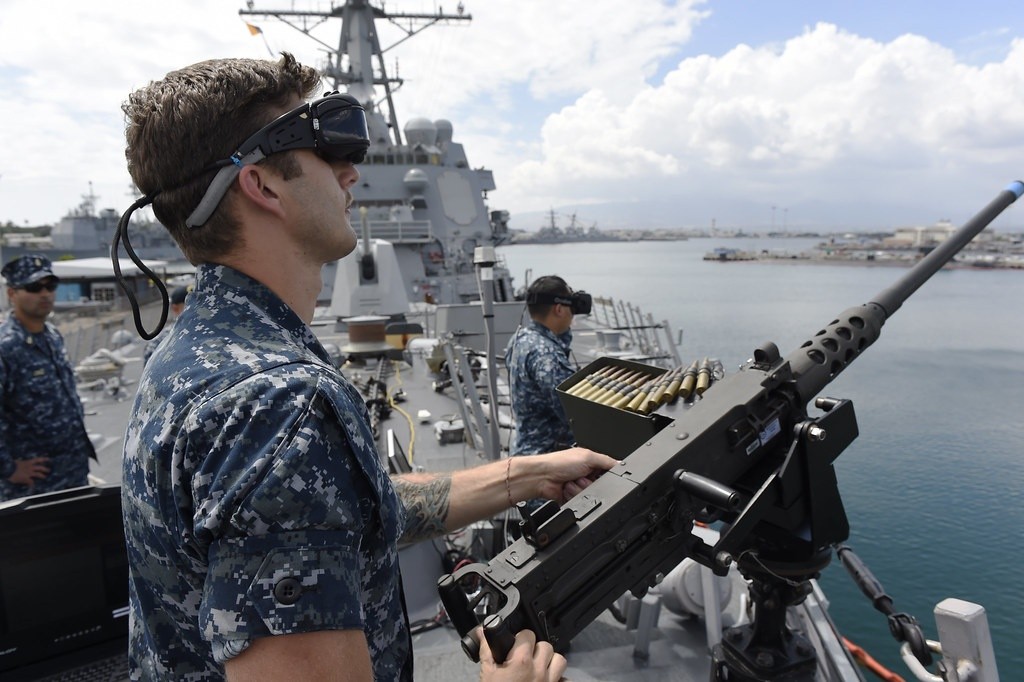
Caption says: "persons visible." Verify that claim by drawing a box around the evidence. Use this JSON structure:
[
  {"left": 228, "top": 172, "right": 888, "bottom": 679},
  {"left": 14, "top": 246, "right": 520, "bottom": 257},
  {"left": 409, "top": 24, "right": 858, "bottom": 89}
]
[
  {"left": 119, "top": 49, "right": 624, "bottom": 682},
  {"left": 506, "top": 274, "right": 574, "bottom": 514},
  {"left": 0, "top": 254, "right": 101, "bottom": 504}
]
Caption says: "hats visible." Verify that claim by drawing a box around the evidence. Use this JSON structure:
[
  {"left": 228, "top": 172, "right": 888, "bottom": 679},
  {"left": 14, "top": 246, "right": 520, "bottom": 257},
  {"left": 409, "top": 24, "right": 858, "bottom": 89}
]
[
  {"left": 2, "top": 255, "right": 58, "bottom": 289},
  {"left": 171, "top": 286, "right": 188, "bottom": 303}
]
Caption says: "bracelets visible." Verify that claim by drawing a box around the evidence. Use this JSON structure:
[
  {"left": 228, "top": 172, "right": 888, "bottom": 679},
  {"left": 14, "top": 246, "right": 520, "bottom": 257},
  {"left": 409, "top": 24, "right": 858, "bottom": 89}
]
[{"left": 506, "top": 458, "right": 514, "bottom": 507}]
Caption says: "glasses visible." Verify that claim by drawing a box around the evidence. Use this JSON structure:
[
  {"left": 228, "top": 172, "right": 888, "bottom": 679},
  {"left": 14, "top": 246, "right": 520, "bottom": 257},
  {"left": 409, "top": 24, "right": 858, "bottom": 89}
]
[
  {"left": 186, "top": 89, "right": 370, "bottom": 230},
  {"left": 16, "top": 280, "right": 57, "bottom": 293}
]
